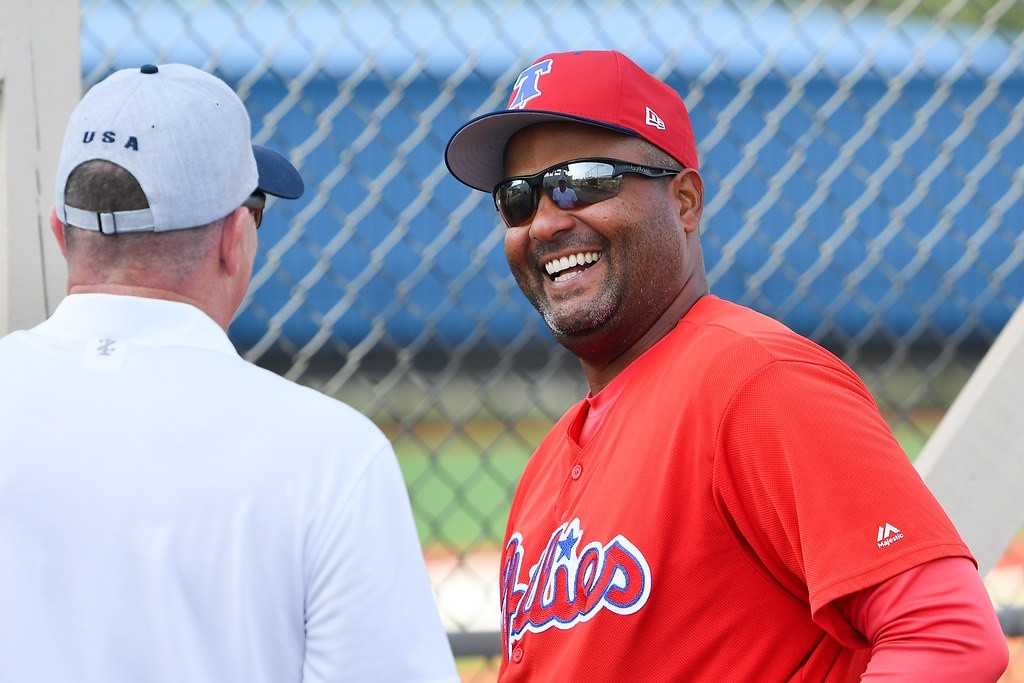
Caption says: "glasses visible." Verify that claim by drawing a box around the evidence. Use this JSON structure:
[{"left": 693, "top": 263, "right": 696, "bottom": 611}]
[
  {"left": 243, "top": 188, "right": 267, "bottom": 227},
  {"left": 493, "top": 156, "right": 683, "bottom": 227},
  {"left": 558, "top": 181, "right": 566, "bottom": 185}
]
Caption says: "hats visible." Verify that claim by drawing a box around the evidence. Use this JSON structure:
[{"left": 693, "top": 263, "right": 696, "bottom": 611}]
[
  {"left": 55, "top": 63, "right": 305, "bottom": 237},
  {"left": 558, "top": 176, "right": 567, "bottom": 183},
  {"left": 444, "top": 50, "right": 698, "bottom": 194}
]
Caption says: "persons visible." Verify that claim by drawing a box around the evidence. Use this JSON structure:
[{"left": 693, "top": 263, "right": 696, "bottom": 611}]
[
  {"left": 0, "top": 62, "right": 463, "bottom": 683},
  {"left": 553, "top": 176, "right": 579, "bottom": 210},
  {"left": 445, "top": 51, "right": 1009, "bottom": 683}
]
